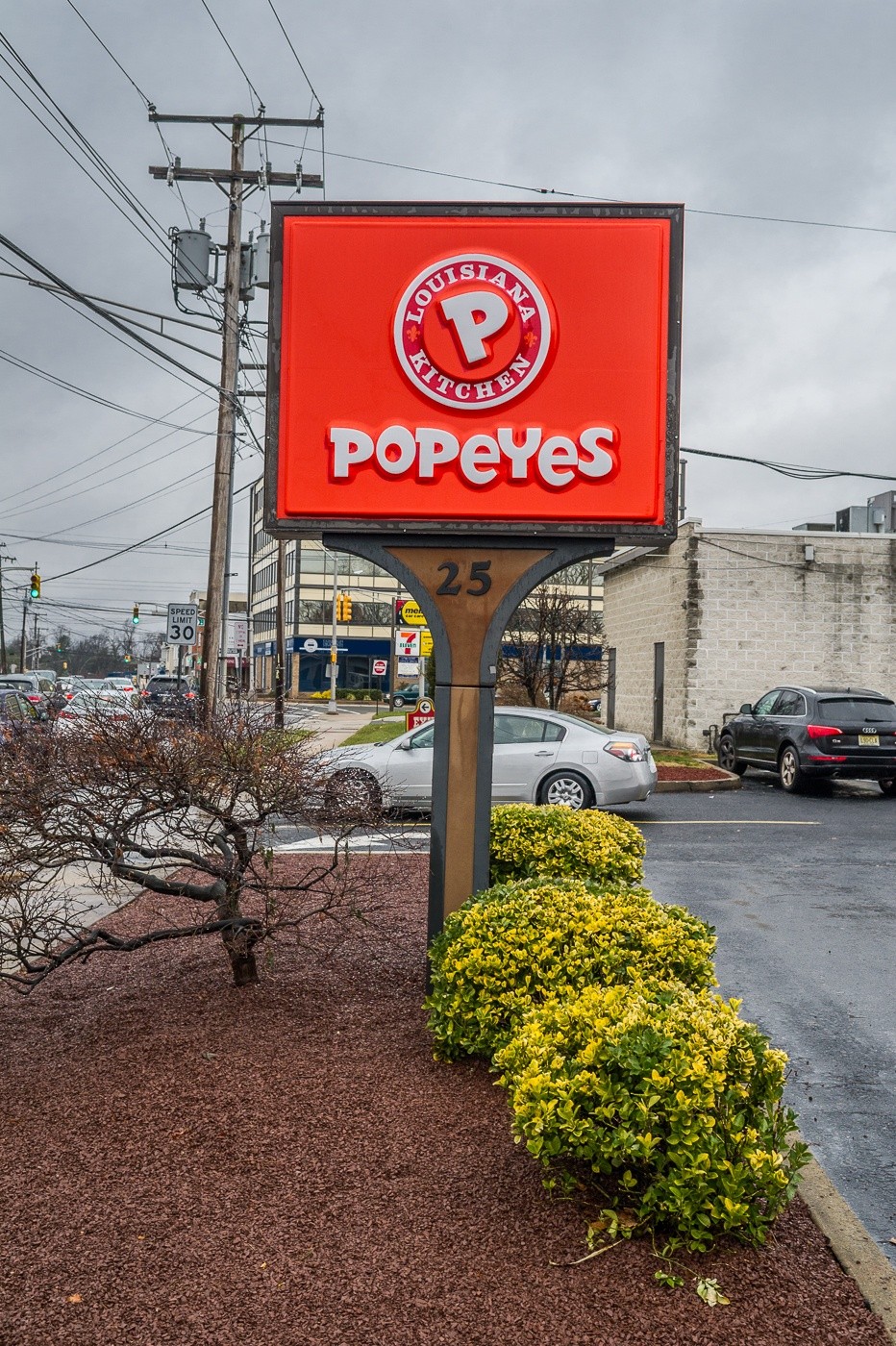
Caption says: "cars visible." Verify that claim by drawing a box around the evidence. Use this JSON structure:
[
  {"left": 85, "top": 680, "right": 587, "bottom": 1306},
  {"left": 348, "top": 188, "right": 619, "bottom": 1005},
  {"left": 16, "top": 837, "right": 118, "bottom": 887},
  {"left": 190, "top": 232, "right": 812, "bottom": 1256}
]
[
  {"left": 299, "top": 704, "right": 656, "bottom": 821},
  {"left": 717, "top": 686, "right": 896, "bottom": 797},
  {"left": 0, "top": 670, "right": 144, "bottom": 745}
]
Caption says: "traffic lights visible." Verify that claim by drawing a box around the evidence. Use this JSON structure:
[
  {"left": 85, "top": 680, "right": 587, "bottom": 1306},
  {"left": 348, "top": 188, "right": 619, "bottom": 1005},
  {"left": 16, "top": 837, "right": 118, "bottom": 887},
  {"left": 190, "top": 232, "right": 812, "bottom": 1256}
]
[
  {"left": 57, "top": 645, "right": 61, "bottom": 653},
  {"left": 124, "top": 655, "right": 130, "bottom": 663},
  {"left": 30, "top": 574, "right": 41, "bottom": 599},
  {"left": 131, "top": 608, "right": 139, "bottom": 624}
]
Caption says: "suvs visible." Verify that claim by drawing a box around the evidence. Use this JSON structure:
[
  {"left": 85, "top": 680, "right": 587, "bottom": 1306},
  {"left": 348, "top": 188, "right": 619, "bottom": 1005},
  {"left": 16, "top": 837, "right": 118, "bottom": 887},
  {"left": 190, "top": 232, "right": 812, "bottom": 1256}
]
[{"left": 143, "top": 675, "right": 196, "bottom": 717}]
